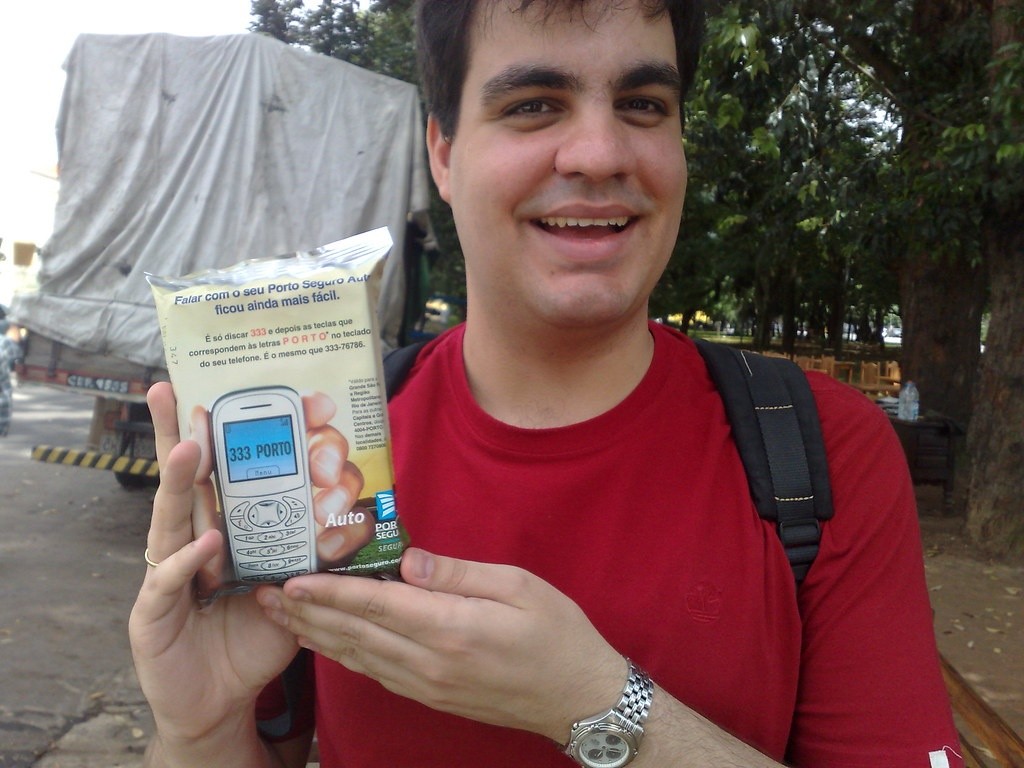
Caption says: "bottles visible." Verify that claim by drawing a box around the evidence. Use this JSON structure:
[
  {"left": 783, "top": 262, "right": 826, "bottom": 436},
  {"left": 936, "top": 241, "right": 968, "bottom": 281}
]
[{"left": 898, "top": 382, "right": 919, "bottom": 420}]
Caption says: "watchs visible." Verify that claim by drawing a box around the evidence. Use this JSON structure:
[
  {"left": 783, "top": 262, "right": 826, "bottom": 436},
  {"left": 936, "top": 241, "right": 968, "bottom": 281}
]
[{"left": 553, "top": 656, "right": 655, "bottom": 768}]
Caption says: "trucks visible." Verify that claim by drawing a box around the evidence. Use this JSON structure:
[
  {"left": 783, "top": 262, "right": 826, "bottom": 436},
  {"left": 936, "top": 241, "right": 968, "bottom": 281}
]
[{"left": 6, "top": 217, "right": 441, "bottom": 490}]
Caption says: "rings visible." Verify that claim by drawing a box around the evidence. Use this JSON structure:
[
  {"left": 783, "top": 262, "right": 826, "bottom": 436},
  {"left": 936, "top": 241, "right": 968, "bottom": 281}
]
[{"left": 145, "top": 537, "right": 193, "bottom": 566}]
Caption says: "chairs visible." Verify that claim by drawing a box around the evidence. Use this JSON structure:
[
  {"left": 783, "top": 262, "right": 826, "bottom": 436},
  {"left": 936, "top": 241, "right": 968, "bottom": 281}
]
[{"left": 760, "top": 349, "right": 903, "bottom": 403}]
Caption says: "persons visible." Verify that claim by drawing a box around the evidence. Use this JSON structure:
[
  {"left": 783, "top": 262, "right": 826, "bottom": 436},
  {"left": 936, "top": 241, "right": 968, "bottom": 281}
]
[
  {"left": 128, "top": 1, "right": 965, "bottom": 768},
  {"left": 0, "top": 320, "right": 23, "bottom": 439}
]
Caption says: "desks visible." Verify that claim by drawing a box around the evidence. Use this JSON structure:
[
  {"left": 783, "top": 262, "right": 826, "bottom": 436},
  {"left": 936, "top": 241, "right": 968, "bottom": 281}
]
[
  {"left": 850, "top": 383, "right": 901, "bottom": 395},
  {"left": 887, "top": 413, "right": 956, "bottom": 519}
]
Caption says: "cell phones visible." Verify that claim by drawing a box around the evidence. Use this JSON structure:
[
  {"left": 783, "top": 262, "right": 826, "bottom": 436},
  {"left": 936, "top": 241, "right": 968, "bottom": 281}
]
[{"left": 210, "top": 386, "right": 325, "bottom": 586}]
